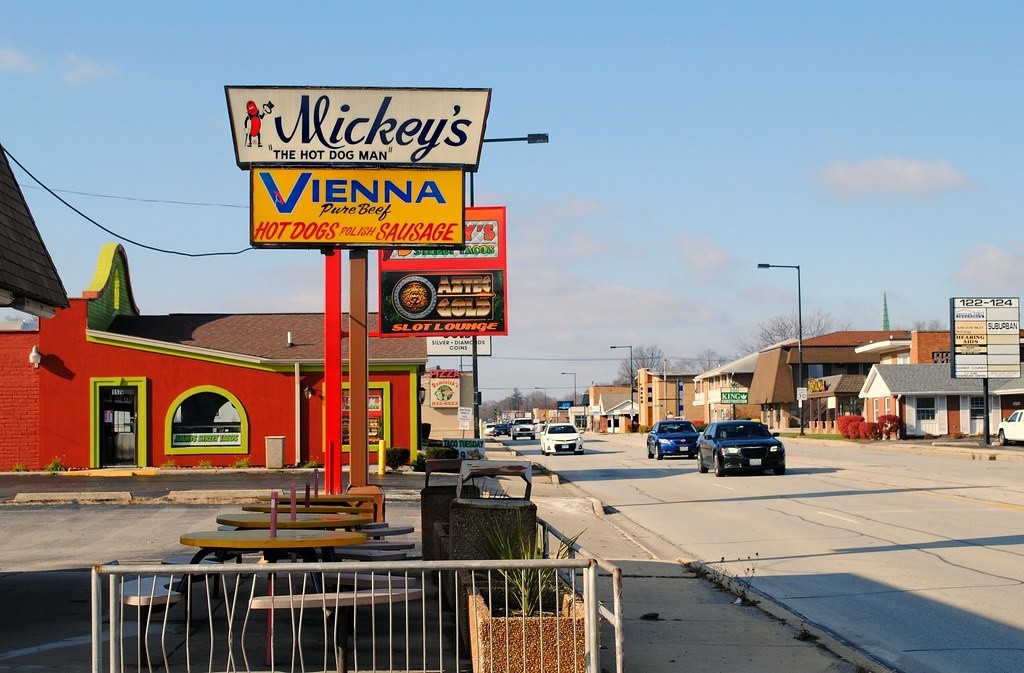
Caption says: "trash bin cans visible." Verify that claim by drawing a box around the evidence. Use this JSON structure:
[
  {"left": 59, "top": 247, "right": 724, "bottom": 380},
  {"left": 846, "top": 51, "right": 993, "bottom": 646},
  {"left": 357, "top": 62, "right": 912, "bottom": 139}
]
[{"left": 265, "top": 436, "right": 286, "bottom": 469}]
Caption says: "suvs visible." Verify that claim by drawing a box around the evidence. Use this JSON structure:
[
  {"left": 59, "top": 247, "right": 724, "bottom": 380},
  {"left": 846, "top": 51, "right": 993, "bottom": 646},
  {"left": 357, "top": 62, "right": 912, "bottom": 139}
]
[
  {"left": 997, "top": 409, "right": 1024, "bottom": 446},
  {"left": 509, "top": 417, "right": 538, "bottom": 440}
]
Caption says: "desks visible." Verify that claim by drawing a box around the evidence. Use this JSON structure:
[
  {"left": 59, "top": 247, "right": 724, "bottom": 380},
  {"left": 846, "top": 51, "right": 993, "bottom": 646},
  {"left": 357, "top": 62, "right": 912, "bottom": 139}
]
[
  {"left": 242, "top": 501, "right": 375, "bottom": 514},
  {"left": 258, "top": 493, "right": 376, "bottom": 505},
  {"left": 149, "top": 529, "right": 368, "bottom": 620},
  {"left": 217, "top": 511, "right": 372, "bottom": 531}
]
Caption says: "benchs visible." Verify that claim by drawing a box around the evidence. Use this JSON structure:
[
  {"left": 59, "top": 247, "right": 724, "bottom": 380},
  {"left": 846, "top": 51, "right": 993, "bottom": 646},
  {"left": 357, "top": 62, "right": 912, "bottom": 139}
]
[{"left": 118, "top": 524, "right": 423, "bottom": 672}]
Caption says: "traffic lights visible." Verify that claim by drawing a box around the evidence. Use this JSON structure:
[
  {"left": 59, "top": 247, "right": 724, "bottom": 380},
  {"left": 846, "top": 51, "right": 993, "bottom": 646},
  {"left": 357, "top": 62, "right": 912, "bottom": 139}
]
[{"left": 494, "top": 408, "right": 497, "bottom": 414}]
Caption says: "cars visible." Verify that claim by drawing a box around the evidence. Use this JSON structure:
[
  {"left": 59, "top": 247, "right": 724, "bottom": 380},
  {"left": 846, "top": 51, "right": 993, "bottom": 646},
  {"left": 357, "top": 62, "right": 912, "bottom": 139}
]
[
  {"left": 494, "top": 424, "right": 511, "bottom": 437},
  {"left": 540, "top": 422, "right": 584, "bottom": 457},
  {"left": 646, "top": 420, "right": 701, "bottom": 460},
  {"left": 483, "top": 423, "right": 497, "bottom": 436},
  {"left": 696, "top": 419, "right": 787, "bottom": 477}
]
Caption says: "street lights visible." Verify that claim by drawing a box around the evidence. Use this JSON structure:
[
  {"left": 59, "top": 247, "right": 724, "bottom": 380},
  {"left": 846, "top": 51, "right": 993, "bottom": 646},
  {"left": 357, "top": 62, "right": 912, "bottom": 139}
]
[
  {"left": 534, "top": 387, "right": 547, "bottom": 409},
  {"left": 758, "top": 263, "right": 805, "bottom": 435},
  {"left": 611, "top": 345, "right": 636, "bottom": 432},
  {"left": 471, "top": 132, "right": 551, "bottom": 436},
  {"left": 561, "top": 372, "right": 577, "bottom": 407}
]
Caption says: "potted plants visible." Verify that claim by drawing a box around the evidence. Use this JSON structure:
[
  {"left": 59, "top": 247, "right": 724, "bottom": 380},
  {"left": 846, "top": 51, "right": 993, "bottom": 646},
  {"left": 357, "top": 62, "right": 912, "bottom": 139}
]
[
  {"left": 425, "top": 446, "right": 461, "bottom": 486},
  {"left": 464, "top": 502, "right": 602, "bottom": 673}
]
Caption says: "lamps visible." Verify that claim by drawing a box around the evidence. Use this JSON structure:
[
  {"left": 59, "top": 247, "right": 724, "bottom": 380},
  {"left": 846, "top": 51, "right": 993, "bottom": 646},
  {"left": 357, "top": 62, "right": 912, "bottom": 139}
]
[{"left": 29, "top": 344, "right": 41, "bottom": 368}]
